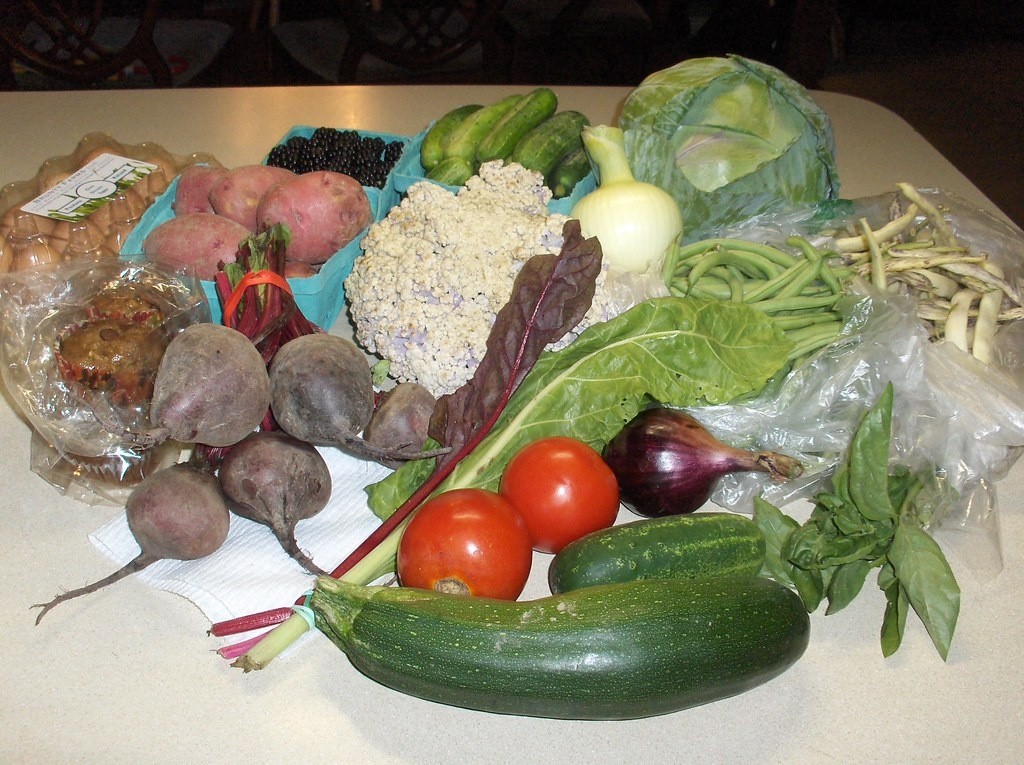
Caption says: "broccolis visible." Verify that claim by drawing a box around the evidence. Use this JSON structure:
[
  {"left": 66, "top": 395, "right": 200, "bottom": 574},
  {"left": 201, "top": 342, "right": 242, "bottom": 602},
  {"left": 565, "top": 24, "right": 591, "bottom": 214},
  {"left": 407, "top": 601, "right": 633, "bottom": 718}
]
[{"left": 343, "top": 160, "right": 609, "bottom": 399}]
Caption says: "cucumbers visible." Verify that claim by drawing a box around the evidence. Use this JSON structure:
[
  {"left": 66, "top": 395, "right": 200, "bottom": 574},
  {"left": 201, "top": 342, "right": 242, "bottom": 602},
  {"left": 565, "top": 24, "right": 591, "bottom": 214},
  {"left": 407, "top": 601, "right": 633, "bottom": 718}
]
[
  {"left": 420, "top": 88, "right": 592, "bottom": 199},
  {"left": 548, "top": 513, "right": 767, "bottom": 596}
]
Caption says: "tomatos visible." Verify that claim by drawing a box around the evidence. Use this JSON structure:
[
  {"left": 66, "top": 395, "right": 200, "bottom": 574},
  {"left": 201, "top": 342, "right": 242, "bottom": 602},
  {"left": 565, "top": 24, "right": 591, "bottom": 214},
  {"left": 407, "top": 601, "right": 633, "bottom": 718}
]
[{"left": 395, "top": 436, "right": 621, "bottom": 601}]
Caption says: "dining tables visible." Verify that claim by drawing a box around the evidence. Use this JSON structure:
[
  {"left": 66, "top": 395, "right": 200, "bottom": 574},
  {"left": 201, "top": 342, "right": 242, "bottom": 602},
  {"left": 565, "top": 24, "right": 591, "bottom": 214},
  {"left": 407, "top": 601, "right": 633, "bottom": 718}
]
[{"left": 0, "top": 84, "right": 1024, "bottom": 765}]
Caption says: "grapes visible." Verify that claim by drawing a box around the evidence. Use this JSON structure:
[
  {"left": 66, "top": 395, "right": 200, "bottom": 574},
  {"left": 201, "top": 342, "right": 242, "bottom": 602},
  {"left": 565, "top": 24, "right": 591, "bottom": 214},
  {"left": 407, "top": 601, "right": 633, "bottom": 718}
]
[{"left": 267, "top": 127, "right": 404, "bottom": 190}]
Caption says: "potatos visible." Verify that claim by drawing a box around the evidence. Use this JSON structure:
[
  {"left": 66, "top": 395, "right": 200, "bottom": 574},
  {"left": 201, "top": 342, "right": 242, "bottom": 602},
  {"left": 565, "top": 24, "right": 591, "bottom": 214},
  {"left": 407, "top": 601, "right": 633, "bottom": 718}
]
[
  {"left": 140, "top": 164, "right": 375, "bottom": 281},
  {"left": 31, "top": 322, "right": 442, "bottom": 627}
]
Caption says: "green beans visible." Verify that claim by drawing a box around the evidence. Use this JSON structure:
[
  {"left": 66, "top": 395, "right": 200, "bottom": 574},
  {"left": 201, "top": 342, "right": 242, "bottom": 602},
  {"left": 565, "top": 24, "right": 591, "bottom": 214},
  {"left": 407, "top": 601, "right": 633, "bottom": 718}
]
[{"left": 664, "top": 184, "right": 1023, "bottom": 366}]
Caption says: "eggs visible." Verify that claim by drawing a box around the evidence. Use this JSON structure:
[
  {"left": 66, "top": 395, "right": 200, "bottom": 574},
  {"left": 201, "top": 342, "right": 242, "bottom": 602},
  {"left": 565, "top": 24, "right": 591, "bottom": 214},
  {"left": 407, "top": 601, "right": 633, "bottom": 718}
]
[{"left": 0, "top": 146, "right": 176, "bottom": 282}]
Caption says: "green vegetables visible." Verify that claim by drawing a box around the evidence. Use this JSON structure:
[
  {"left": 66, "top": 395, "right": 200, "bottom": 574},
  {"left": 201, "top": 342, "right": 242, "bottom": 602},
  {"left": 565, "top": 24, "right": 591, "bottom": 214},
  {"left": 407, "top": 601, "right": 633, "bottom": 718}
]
[
  {"left": 756, "top": 380, "right": 962, "bottom": 660},
  {"left": 236, "top": 300, "right": 798, "bottom": 686}
]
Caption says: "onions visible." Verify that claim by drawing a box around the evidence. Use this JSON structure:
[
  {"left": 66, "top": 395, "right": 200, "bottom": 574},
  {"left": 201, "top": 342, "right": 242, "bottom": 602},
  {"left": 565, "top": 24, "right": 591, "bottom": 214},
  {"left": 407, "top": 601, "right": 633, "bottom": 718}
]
[{"left": 602, "top": 409, "right": 804, "bottom": 521}]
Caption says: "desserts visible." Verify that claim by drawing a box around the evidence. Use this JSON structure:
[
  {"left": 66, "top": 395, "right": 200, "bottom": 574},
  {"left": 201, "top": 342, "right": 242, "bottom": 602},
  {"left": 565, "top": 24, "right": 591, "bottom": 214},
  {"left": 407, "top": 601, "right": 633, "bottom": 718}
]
[{"left": 59, "top": 289, "right": 173, "bottom": 380}]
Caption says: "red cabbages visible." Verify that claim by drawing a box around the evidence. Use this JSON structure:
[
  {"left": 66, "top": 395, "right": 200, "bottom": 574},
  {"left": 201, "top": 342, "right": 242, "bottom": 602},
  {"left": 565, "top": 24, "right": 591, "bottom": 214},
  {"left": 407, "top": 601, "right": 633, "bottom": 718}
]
[{"left": 618, "top": 53, "right": 839, "bottom": 234}]
